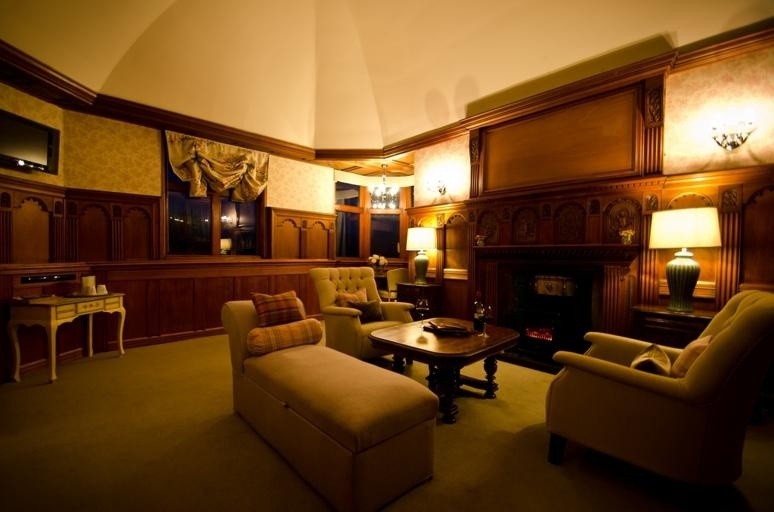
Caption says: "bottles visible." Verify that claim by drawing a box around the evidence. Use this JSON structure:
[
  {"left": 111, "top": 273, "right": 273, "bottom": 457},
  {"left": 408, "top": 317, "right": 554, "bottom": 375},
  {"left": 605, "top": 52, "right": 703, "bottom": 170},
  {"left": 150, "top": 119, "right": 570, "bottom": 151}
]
[{"left": 472, "top": 289, "right": 484, "bottom": 331}]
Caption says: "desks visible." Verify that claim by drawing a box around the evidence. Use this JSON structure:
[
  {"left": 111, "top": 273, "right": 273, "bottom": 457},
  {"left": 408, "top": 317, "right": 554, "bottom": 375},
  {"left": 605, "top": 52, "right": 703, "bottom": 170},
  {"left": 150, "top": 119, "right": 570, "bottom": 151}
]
[
  {"left": 628, "top": 304, "right": 715, "bottom": 349},
  {"left": 6, "top": 292, "right": 127, "bottom": 384},
  {"left": 396, "top": 283, "right": 442, "bottom": 321}
]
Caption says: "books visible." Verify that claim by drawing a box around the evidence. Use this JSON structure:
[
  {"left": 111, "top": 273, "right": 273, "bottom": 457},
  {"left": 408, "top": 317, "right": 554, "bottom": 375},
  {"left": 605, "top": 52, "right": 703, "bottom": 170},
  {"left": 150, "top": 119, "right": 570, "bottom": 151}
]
[
  {"left": 423, "top": 316, "right": 471, "bottom": 337},
  {"left": 428, "top": 318, "right": 466, "bottom": 331}
]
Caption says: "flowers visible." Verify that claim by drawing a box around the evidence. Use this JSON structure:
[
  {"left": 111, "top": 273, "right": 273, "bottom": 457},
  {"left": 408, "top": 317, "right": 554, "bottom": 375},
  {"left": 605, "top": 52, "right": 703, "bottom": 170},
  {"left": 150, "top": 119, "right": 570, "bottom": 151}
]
[{"left": 368, "top": 254, "right": 388, "bottom": 267}]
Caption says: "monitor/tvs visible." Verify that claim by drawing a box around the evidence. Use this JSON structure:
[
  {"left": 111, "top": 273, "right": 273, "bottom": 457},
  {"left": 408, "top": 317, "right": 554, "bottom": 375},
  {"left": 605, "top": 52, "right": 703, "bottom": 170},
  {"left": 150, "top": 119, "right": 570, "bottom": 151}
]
[{"left": 0, "top": 108, "right": 60, "bottom": 175}]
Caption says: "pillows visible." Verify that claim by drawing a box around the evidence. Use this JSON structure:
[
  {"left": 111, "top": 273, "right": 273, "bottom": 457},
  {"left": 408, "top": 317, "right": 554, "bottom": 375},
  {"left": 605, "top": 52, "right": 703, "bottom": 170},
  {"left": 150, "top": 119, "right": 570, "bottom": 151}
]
[
  {"left": 334, "top": 287, "right": 369, "bottom": 307},
  {"left": 671, "top": 334, "right": 712, "bottom": 378},
  {"left": 630, "top": 343, "right": 671, "bottom": 376},
  {"left": 346, "top": 300, "right": 384, "bottom": 324},
  {"left": 250, "top": 290, "right": 304, "bottom": 327},
  {"left": 247, "top": 318, "right": 323, "bottom": 356}
]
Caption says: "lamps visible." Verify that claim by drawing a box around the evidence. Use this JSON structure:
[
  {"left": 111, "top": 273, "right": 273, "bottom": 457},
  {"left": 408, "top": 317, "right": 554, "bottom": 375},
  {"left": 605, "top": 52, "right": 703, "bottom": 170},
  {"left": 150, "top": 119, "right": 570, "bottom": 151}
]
[
  {"left": 649, "top": 207, "right": 722, "bottom": 312},
  {"left": 367, "top": 164, "right": 400, "bottom": 209},
  {"left": 406, "top": 227, "right": 436, "bottom": 285}
]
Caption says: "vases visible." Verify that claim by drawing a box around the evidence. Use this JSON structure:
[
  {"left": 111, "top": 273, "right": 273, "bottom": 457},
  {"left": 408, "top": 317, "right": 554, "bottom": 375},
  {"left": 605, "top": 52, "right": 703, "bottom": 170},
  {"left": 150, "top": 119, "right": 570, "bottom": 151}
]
[{"left": 376, "top": 265, "right": 384, "bottom": 273}]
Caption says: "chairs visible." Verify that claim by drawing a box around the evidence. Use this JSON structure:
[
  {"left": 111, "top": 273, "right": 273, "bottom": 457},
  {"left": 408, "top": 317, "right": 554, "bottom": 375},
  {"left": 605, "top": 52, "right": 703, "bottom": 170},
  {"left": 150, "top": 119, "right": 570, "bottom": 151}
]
[
  {"left": 310, "top": 267, "right": 416, "bottom": 364},
  {"left": 220, "top": 298, "right": 439, "bottom": 512},
  {"left": 544, "top": 289, "right": 774, "bottom": 503},
  {"left": 378, "top": 268, "right": 407, "bottom": 302}
]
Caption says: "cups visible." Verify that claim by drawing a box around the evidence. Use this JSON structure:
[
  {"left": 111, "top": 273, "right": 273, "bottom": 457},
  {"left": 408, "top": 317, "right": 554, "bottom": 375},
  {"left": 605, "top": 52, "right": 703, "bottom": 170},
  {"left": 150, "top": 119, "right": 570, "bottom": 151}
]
[
  {"left": 80, "top": 275, "right": 106, "bottom": 296},
  {"left": 534, "top": 276, "right": 574, "bottom": 297}
]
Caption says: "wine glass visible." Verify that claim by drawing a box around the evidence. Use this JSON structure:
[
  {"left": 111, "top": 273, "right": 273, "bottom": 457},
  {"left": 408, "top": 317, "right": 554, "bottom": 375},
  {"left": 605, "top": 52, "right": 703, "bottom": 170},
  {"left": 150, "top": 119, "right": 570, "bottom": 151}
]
[
  {"left": 415, "top": 298, "right": 428, "bottom": 331},
  {"left": 476, "top": 304, "right": 492, "bottom": 339}
]
